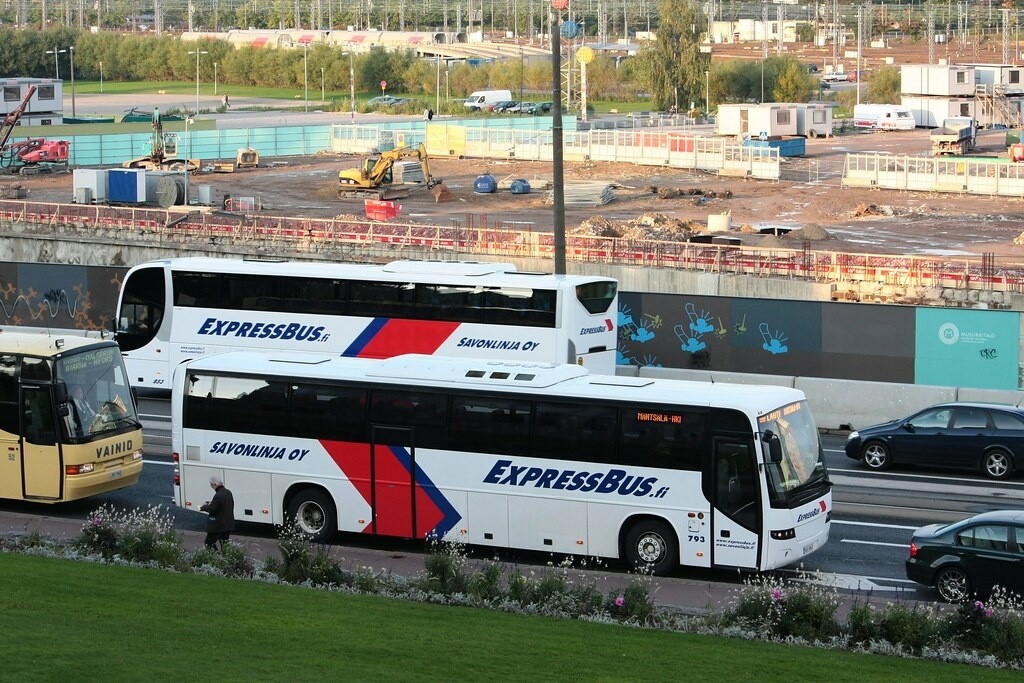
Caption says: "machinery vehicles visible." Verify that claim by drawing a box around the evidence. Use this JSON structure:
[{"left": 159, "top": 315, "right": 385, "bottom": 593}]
[
  {"left": 122, "top": 105, "right": 195, "bottom": 174},
  {"left": 0, "top": 86, "right": 72, "bottom": 178},
  {"left": 336, "top": 142, "right": 454, "bottom": 204}
]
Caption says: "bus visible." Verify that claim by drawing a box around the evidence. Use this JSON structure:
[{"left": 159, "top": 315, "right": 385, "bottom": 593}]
[
  {"left": 171, "top": 345, "right": 836, "bottom": 578},
  {"left": 0, "top": 324, "right": 144, "bottom": 504},
  {"left": 109, "top": 256, "right": 618, "bottom": 399}
]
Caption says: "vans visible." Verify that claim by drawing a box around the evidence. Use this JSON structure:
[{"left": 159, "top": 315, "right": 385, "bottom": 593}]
[
  {"left": 463, "top": 90, "right": 513, "bottom": 113},
  {"left": 853, "top": 103, "right": 916, "bottom": 132}
]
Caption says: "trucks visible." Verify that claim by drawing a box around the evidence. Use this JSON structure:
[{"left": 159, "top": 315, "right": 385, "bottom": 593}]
[{"left": 929, "top": 113, "right": 979, "bottom": 157}]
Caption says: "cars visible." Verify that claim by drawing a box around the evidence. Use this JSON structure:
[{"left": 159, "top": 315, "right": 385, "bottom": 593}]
[
  {"left": 505, "top": 101, "right": 537, "bottom": 115},
  {"left": 847, "top": 70, "right": 868, "bottom": 82},
  {"left": 819, "top": 80, "right": 831, "bottom": 91},
  {"left": 823, "top": 71, "right": 848, "bottom": 82},
  {"left": 527, "top": 101, "right": 567, "bottom": 116},
  {"left": 493, "top": 100, "right": 520, "bottom": 115},
  {"left": 905, "top": 509, "right": 1024, "bottom": 605},
  {"left": 482, "top": 101, "right": 503, "bottom": 113},
  {"left": 844, "top": 401, "right": 1024, "bottom": 481},
  {"left": 803, "top": 63, "right": 818, "bottom": 74}
]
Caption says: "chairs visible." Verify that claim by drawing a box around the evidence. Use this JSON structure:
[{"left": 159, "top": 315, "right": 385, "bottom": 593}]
[
  {"left": 960, "top": 527, "right": 1004, "bottom": 548},
  {"left": 242, "top": 384, "right": 701, "bottom": 462}
]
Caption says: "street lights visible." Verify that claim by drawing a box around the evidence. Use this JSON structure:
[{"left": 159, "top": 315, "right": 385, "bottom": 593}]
[
  {"left": 46, "top": 47, "right": 67, "bottom": 79},
  {"left": 320, "top": 67, "right": 325, "bottom": 101},
  {"left": 444, "top": 70, "right": 449, "bottom": 102},
  {"left": 184, "top": 117, "right": 195, "bottom": 207},
  {"left": 213, "top": 62, "right": 219, "bottom": 94},
  {"left": 849, "top": 2, "right": 869, "bottom": 105},
  {"left": 99, "top": 61, "right": 103, "bottom": 94},
  {"left": 342, "top": 51, "right": 355, "bottom": 118},
  {"left": 704, "top": 71, "right": 711, "bottom": 114},
  {"left": 188, "top": 47, "right": 208, "bottom": 118}
]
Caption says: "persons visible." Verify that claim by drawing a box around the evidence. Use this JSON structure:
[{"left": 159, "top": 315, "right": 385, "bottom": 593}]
[
  {"left": 222, "top": 95, "right": 230, "bottom": 107},
  {"left": 738, "top": 449, "right": 756, "bottom": 487},
  {"left": 200, "top": 477, "right": 235, "bottom": 552},
  {"left": 423, "top": 107, "right": 434, "bottom": 121},
  {"left": 67, "top": 386, "right": 95, "bottom": 426}
]
